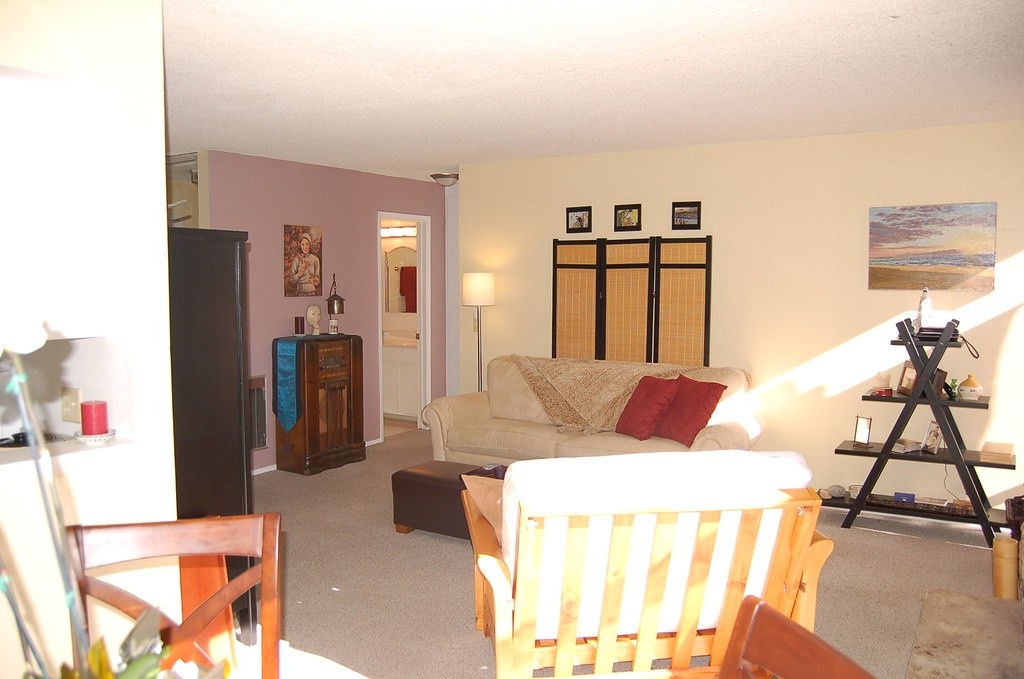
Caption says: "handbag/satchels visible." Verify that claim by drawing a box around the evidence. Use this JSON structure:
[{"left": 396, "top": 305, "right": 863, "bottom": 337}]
[{"left": 918, "top": 327, "right": 979, "bottom": 359}]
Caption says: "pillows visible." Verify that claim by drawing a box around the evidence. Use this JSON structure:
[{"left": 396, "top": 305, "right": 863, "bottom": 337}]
[
  {"left": 615, "top": 376, "right": 680, "bottom": 442},
  {"left": 652, "top": 373, "right": 728, "bottom": 448}
]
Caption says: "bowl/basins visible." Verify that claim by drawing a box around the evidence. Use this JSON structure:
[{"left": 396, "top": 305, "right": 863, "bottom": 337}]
[{"left": 74, "top": 428, "right": 115, "bottom": 444}]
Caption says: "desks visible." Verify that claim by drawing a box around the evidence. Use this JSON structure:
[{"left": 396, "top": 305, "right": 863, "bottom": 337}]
[
  {"left": 903, "top": 589, "right": 1024, "bottom": 679},
  {"left": 270, "top": 332, "right": 365, "bottom": 476}
]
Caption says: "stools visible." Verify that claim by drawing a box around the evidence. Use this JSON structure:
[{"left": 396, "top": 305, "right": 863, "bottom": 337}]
[{"left": 392, "top": 461, "right": 482, "bottom": 540}]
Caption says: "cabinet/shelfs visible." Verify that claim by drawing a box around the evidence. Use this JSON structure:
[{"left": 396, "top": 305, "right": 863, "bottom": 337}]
[
  {"left": 818, "top": 316, "right": 1017, "bottom": 548},
  {"left": 168, "top": 226, "right": 256, "bottom": 647},
  {"left": 382, "top": 346, "right": 418, "bottom": 423}
]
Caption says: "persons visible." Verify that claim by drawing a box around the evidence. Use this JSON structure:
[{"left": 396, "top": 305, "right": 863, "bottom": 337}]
[
  {"left": 925, "top": 425, "right": 939, "bottom": 450},
  {"left": 306, "top": 305, "right": 322, "bottom": 328}
]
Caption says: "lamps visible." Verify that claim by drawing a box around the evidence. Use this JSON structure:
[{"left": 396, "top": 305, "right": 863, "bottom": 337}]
[
  {"left": 325, "top": 273, "right": 345, "bottom": 319},
  {"left": 430, "top": 173, "right": 459, "bottom": 186},
  {"left": 462, "top": 271, "right": 496, "bottom": 391}
]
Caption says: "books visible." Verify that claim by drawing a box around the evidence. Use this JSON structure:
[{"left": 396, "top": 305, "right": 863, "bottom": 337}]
[{"left": 980, "top": 441, "right": 1014, "bottom": 464}]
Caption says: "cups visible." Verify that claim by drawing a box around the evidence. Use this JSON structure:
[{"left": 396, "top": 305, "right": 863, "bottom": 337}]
[
  {"left": 994, "top": 537, "right": 1018, "bottom": 600},
  {"left": 328, "top": 319, "right": 338, "bottom": 334},
  {"left": 877, "top": 372, "right": 891, "bottom": 388}
]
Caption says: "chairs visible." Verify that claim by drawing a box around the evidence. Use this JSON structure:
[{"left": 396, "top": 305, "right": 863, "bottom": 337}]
[
  {"left": 64, "top": 510, "right": 282, "bottom": 679},
  {"left": 718, "top": 594, "right": 875, "bottom": 679},
  {"left": 459, "top": 449, "right": 837, "bottom": 679}
]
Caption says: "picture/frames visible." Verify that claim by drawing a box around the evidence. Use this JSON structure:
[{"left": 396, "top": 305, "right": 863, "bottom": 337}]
[
  {"left": 922, "top": 420, "right": 941, "bottom": 454},
  {"left": 566, "top": 206, "right": 592, "bottom": 234},
  {"left": 671, "top": 201, "right": 701, "bottom": 230},
  {"left": 896, "top": 360, "right": 917, "bottom": 396},
  {"left": 932, "top": 368, "right": 953, "bottom": 398},
  {"left": 613, "top": 203, "right": 642, "bottom": 232}
]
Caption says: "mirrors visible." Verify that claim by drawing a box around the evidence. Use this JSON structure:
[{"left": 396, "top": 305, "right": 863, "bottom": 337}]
[{"left": 384, "top": 246, "right": 417, "bottom": 314}]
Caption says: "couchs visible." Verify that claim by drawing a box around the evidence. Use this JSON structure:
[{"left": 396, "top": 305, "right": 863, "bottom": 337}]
[{"left": 420, "top": 354, "right": 763, "bottom": 466}]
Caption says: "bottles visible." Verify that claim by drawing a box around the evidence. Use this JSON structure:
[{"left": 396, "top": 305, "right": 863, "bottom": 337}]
[{"left": 958, "top": 374, "right": 982, "bottom": 400}]
